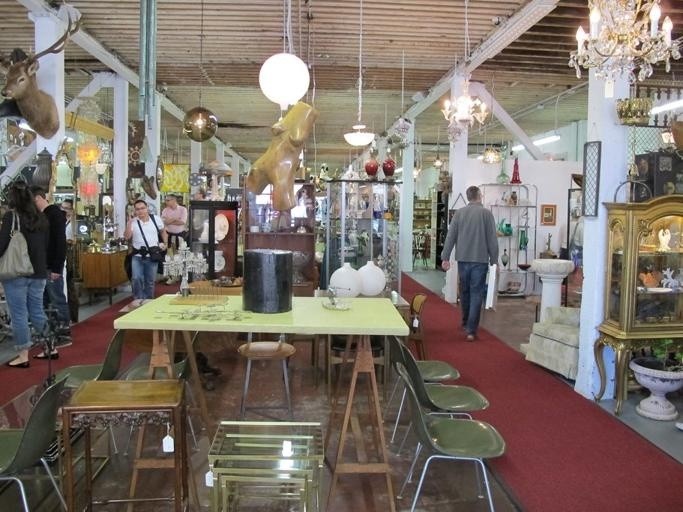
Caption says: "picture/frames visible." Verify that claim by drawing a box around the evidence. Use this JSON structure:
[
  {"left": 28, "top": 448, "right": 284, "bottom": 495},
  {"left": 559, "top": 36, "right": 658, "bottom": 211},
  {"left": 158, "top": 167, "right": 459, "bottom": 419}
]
[{"left": 540, "top": 205, "right": 557, "bottom": 226}]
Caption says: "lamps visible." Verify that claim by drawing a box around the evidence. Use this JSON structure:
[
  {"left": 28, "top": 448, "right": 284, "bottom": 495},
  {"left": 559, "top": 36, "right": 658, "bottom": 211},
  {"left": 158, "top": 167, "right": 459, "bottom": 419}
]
[
  {"left": 343, "top": 0, "right": 375, "bottom": 148},
  {"left": 439, "top": 0, "right": 490, "bottom": 128},
  {"left": 258, "top": 0, "right": 310, "bottom": 112},
  {"left": 559, "top": 0, "right": 683, "bottom": 83},
  {"left": 432, "top": 125, "right": 445, "bottom": 169},
  {"left": 477, "top": 140, "right": 501, "bottom": 164}
]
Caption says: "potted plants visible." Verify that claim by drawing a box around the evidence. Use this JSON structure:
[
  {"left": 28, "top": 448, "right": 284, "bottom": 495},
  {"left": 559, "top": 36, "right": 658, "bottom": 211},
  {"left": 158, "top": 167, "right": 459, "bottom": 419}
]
[{"left": 627, "top": 339, "right": 683, "bottom": 421}]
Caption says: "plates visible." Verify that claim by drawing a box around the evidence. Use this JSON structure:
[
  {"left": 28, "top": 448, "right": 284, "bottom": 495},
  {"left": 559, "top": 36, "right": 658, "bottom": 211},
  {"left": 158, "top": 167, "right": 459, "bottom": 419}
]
[
  {"left": 214, "top": 214, "right": 230, "bottom": 241},
  {"left": 212, "top": 278, "right": 242, "bottom": 287},
  {"left": 198, "top": 239, "right": 210, "bottom": 244}
]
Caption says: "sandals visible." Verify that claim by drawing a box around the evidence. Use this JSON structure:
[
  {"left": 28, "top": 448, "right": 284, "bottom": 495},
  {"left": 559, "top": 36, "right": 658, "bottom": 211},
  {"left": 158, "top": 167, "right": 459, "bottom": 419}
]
[{"left": 464, "top": 332, "right": 479, "bottom": 342}]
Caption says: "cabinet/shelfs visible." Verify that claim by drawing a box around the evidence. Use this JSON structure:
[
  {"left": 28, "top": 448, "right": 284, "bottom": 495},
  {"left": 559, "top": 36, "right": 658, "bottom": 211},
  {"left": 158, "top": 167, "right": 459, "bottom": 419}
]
[
  {"left": 478, "top": 181, "right": 536, "bottom": 297},
  {"left": 189, "top": 200, "right": 238, "bottom": 283},
  {"left": 414, "top": 199, "right": 432, "bottom": 229},
  {"left": 327, "top": 178, "right": 403, "bottom": 293}
]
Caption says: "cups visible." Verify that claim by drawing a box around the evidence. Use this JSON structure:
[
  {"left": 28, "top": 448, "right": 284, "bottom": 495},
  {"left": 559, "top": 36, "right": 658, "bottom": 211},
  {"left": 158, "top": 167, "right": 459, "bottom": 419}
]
[
  {"left": 262, "top": 223, "right": 273, "bottom": 234},
  {"left": 323, "top": 285, "right": 353, "bottom": 312},
  {"left": 250, "top": 226, "right": 260, "bottom": 233}
]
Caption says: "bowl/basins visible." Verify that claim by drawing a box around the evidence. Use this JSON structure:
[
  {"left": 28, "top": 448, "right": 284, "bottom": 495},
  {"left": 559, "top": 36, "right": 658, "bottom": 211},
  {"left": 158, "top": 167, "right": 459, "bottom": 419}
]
[{"left": 517, "top": 264, "right": 530, "bottom": 271}]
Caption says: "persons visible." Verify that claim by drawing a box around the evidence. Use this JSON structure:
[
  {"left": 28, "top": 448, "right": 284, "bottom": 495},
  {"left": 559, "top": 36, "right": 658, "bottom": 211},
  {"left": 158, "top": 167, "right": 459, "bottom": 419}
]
[
  {"left": 175, "top": 195, "right": 186, "bottom": 207},
  {"left": 60, "top": 199, "right": 79, "bottom": 325},
  {"left": 441, "top": 186, "right": 500, "bottom": 342},
  {"left": 184, "top": 187, "right": 208, "bottom": 243},
  {"left": 159, "top": 193, "right": 187, "bottom": 285},
  {"left": 30, "top": 184, "right": 73, "bottom": 348},
  {"left": 122, "top": 200, "right": 169, "bottom": 301},
  {"left": 0, "top": 179, "right": 58, "bottom": 369}
]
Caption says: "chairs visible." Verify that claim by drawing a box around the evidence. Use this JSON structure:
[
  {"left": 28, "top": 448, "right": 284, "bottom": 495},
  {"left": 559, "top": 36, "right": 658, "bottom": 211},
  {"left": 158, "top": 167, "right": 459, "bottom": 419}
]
[
  {"left": 124, "top": 331, "right": 199, "bottom": 456},
  {"left": 0, "top": 373, "right": 78, "bottom": 511},
  {"left": 53, "top": 328, "right": 124, "bottom": 455},
  {"left": 395, "top": 337, "right": 491, "bottom": 483},
  {"left": 386, "top": 333, "right": 460, "bottom": 442},
  {"left": 393, "top": 361, "right": 508, "bottom": 512},
  {"left": 236, "top": 332, "right": 296, "bottom": 420},
  {"left": 525, "top": 306, "right": 580, "bottom": 380}
]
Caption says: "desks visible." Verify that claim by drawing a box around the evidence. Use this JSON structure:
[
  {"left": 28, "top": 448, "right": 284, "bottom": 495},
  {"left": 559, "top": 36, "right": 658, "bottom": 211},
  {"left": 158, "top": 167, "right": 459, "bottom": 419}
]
[
  {"left": 63, "top": 378, "right": 189, "bottom": 512},
  {"left": 114, "top": 294, "right": 410, "bottom": 512},
  {"left": 80, "top": 244, "right": 130, "bottom": 306}
]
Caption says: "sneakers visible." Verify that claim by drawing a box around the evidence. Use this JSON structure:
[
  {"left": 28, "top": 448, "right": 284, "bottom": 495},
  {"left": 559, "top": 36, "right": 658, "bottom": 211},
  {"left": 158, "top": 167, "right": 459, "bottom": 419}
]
[
  {"left": 43, "top": 337, "right": 73, "bottom": 349},
  {"left": 165, "top": 275, "right": 181, "bottom": 285}
]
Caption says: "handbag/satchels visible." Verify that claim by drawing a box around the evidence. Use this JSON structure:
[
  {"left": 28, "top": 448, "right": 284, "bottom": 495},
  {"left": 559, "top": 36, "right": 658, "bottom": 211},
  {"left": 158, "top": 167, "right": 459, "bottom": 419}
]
[
  {"left": 0, "top": 209, "right": 35, "bottom": 282},
  {"left": 148, "top": 246, "right": 166, "bottom": 263}
]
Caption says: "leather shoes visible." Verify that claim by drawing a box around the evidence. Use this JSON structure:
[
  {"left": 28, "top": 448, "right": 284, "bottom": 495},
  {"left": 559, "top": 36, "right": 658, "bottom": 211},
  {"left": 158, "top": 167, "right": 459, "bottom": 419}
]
[
  {"left": 5, "top": 360, "right": 31, "bottom": 368},
  {"left": 32, "top": 349, "right": 59, "bottom": 360}
]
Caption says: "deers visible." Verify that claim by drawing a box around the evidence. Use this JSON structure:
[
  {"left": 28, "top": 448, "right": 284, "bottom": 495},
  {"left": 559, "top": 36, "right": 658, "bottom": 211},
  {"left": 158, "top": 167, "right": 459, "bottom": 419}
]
[{"left": 0, "top": 10, "right": 85, "bottom": 140}]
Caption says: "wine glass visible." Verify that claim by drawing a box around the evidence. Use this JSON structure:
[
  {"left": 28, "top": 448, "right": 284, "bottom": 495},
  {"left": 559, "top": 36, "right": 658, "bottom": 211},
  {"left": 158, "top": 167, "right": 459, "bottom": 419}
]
[{"left": 292, "top": 251, "right": 313, "bottom": 285}]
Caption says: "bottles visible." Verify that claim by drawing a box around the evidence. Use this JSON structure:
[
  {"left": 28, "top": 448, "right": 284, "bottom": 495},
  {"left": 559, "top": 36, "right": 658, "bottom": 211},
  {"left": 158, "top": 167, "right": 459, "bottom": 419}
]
[
  {"left": 359, "top": 261, "right": 387, "bottom": 297},
  {"left": 511, "top": 156, "right": 522, "bottom": 184},
  {"left": 383, "top": 148, "right": 396, "bottom": 180},
  {"left": 365, "top": 148, "right": 379, "bottom": 177},
  {"left": 511, "top": 191, "right": 517, "bottom": 205},
  {"left": 496, "top": 158, "right": 510, "bottom": 184},
  {"left": 214, "top": 251, "right": 226, "bottom": 272},
  {"left": 330, "top": 262, "right": 360, "bottom": 297},
  {"left": 504, "top": 223, "right": 513, "bottom": 235},
  {"left": 520, "top": 229, "right": 527, "bottom": 250},
  {"left": 198, "top": 222, "right": 209, "bottom": 238}
]
